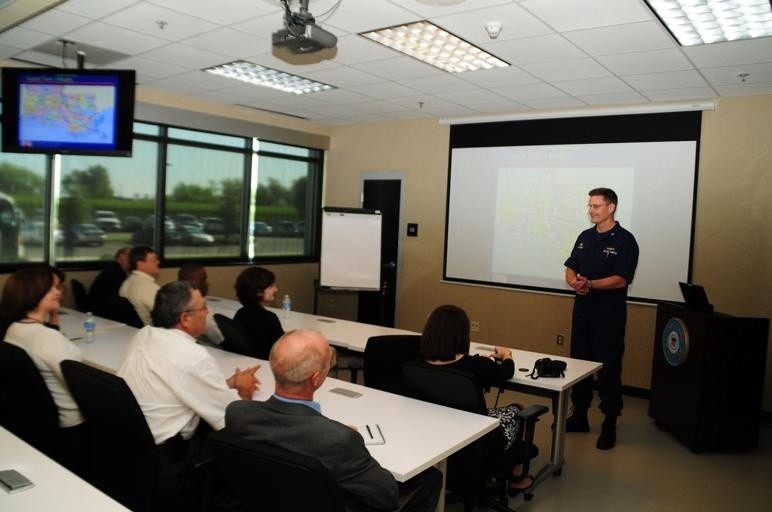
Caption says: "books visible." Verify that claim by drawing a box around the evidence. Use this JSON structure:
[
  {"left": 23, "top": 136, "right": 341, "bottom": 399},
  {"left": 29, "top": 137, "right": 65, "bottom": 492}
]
[{"left": 352, "top": 423, "right": 385, "bottom": 446}]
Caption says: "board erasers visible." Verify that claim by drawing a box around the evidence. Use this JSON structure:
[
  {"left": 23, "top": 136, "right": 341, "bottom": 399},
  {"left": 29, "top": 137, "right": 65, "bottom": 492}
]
[{"left": 321, "top": 286, "right": 331, "bottom": 289}]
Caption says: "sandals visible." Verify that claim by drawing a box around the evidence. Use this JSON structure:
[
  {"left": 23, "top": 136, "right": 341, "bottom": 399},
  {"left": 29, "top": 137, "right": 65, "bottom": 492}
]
[{"left": 507, "top": 474, "right": 535, "bottom": 498}]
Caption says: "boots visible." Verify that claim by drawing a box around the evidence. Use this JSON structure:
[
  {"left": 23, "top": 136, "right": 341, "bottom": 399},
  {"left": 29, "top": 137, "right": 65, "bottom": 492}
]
[
  {"left": 596, "top": 414, "right": 618, "bottom": 450},
  {"left": 551, "top": 401, "right": 590, "bottom": 433}
]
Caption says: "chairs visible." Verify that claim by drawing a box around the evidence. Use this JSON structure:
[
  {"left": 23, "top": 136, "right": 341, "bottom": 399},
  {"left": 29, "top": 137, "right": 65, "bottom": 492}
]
[
  {"left": 60, "top": 357, "right": 183, "bottom": 511},
  {"left": 96, "top": 293, "right": 145, "bottom": 328},
  {"left": 211, "top": 431, "right": 340, "bottom": 511},
  {"left": 357, "top": 329, "right": 429, "bottom": 392},
  {"left": 399, "top": 366, "right": 548, "bottom": 505},
  {"left": 2, "top": 342, "right": 63, "bottom": 459},
  {"left": 213, "top": 313, "right": 267, "bottom": 357},
  {"left": 69, "top": 280, "right": 93, "bottom": 313}
]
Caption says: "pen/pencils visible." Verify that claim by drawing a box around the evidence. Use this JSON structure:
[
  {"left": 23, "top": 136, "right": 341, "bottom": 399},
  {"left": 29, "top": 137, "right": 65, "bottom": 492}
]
[
  {"left": 366, "top": 425, "right": 374, "bottom": 439},
  {"left": 69, "top": 337, "right": 82, "bottom": 341}
]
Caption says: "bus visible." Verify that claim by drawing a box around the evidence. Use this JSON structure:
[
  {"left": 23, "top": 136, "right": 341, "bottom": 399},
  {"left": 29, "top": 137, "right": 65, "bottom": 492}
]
[{"left": 0, "top": 193, "right": 23, "bottom": 260}]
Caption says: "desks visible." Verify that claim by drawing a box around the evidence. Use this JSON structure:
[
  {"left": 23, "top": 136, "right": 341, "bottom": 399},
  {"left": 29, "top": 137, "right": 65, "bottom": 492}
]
[
  {"left": 1, "top": 424, "right": 131, "bottom": 511},
  {"left": 158, "top": 283, "right": 603, "bottom": 504},
  {"left": 44, "top": 306, "right": 500, "bottom": 511}
]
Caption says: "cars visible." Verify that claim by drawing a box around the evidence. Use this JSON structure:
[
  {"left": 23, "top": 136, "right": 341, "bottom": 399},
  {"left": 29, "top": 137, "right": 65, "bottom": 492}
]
[{"left": 17, "top": 209, "right": 308, "bottom": 248}]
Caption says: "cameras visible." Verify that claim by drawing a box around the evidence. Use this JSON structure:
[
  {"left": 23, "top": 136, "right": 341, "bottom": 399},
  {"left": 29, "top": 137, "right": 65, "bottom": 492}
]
[{"left": 534, "top": 356, "right": 567, "bottom": 378}]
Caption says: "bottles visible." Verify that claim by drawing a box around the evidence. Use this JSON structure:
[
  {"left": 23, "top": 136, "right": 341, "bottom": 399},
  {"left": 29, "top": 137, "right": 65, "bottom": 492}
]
[
  {"left": 83, "top": 312, "right": 96, "bottom": 342},
  {"left": 282, "top": 294, "right": 291, "bottom": 320}
]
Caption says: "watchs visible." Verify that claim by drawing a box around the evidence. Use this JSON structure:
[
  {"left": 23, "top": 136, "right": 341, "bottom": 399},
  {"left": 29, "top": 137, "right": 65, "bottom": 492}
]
[{"left": 587, "top": 279, "right": 592, "bottom": 290}]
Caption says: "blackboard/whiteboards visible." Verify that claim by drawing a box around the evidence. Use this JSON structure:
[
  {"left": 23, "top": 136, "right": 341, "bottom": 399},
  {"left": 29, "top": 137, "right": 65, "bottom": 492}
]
[{"left": 319, "top": 206, "right": 382, "bottom": 292}]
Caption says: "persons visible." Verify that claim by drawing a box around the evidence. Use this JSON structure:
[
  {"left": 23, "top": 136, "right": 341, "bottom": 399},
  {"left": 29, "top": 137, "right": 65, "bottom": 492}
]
[
  {"left": 85, "top": 246, "right": 131, "bottom": 301},
  {"left": 224, "top": 264, "right": 285, "bottom": 361},
  {"left": 174, "top": 260, "right": 227, "bottom": 349},
  {"left": 224, "top": 326, "right": 445, "bottom": 510},
  {"left": 550, "top": 187, "right": 640, "bottom": 451},
  {"left": 112, "top": 278, "right": 262, "bottom": 501},
  {"left": 2, "top": 263, "right": 90, "bottom": 430},
  {"left": 42, "top": 265, "right": 65, "bottom": 331},
  {"left": 398, "top": 301, "right": 536, "bottom": 500},
  {"left": 118, "top": 245, "right": 164, "bottom": 326}
]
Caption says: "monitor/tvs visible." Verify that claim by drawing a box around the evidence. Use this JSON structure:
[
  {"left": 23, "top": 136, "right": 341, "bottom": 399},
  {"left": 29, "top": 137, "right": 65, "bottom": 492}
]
[{"left": 0, "top": 68, "right": 138, "bottom": 159}]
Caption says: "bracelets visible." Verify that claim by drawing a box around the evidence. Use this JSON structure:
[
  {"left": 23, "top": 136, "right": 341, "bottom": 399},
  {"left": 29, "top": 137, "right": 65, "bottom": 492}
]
[{"left": 501, "top": 351, "right": 513, "bottom": 358}]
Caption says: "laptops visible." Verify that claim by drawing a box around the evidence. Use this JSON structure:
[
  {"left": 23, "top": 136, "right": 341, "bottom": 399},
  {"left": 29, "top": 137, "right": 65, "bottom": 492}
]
[{"left": 678, "top": 281, "right": 712, "bottom": 310}]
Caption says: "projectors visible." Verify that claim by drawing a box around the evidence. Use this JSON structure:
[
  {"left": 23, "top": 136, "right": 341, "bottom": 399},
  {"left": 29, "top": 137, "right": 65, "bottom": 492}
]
[{"left": 271, "top": 24, "right": 338, "bottom": 54}]
